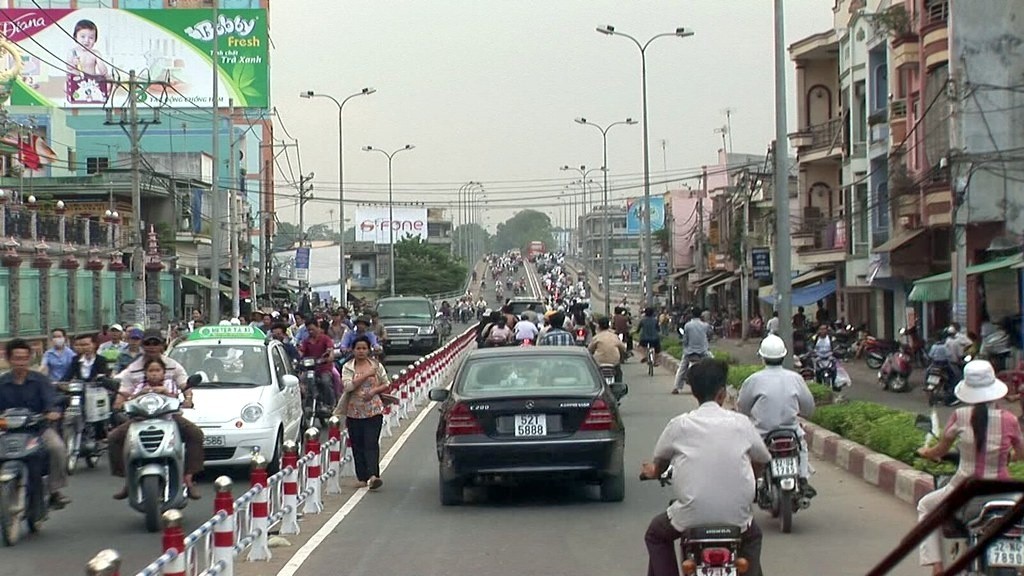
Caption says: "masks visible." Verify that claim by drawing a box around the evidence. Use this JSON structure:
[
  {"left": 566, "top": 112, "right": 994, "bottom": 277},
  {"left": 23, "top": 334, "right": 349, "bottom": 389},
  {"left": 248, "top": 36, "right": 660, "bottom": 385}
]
[{"left": 51, "top": 337, "right": 65, "bottom": 347}]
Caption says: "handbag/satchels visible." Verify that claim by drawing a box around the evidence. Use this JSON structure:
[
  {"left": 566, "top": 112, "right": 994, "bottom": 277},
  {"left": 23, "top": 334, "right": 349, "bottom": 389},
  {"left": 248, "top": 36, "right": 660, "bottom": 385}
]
[{"left": 834, "top": 361, "right": 852, "bottom": 388}]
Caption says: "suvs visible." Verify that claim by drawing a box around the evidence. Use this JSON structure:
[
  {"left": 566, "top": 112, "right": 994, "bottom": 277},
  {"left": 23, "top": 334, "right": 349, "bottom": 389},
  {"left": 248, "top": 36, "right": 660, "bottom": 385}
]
[{"left": 374, "top": 294, "right": 443, "bottom": 357}]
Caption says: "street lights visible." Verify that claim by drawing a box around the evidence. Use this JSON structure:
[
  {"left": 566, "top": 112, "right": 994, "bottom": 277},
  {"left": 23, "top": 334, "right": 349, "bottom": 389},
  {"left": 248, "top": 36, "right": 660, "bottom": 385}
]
[
  {"left": 299, "top": 86, "right": 377, "bottom": 315},
  {"left": 574, "top": 117, "right": 639, "bottom": 322},
  {"left": 554, "top": 165, "right": 610, "bottom": 291},
  {"left": 595, "top": 22, "right": 694, "bottom": 314},
  {"left": 457, "top": 180, "right": 488, "bottom": 279},
  {"left": 362, "top": 143, "right": 417, "bottom": 296}
]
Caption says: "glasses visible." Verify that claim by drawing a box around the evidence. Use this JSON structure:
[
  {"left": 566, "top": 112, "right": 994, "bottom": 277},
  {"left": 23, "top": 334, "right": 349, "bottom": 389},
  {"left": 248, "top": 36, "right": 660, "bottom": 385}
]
[{"left": 141, "top": 339, "right": 164, "bottom": 347}]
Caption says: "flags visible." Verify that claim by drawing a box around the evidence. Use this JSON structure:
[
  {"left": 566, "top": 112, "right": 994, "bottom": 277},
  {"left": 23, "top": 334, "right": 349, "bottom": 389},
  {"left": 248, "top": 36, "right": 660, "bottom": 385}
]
[{"left": 18, "top": 132, "right": 58, "bottom": 170}]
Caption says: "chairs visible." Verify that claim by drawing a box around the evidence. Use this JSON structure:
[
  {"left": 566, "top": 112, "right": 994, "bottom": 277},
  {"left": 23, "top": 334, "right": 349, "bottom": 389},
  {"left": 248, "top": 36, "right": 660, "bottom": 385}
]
[
  {"left": 242, "top": 351, "right": 262, "bottom": 374},
  {"left": 477, "top": 365, "right": 500, "bottom": 385},
  {"left": 184, "top": 349, "right": 205, "bottom": 370},
  {"left": 202, "top": 358, "right": 223, "bottom": 381},
  {"left": 549, "top": 364, "right": 581, "bottom": 385}
]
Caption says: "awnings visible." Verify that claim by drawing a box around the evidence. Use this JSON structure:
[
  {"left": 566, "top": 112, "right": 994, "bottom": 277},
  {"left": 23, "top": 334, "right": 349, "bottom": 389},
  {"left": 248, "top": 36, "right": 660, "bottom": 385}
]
[
  {"left": 909, "top": 253, "right": 1024, "bottom": 301},
  {"left": 756, "top": 267, "right": 836, "bottom": 307},
  {"left": 669, "top": 267, "right": 740, "bottom": 294},
  {"left": 181, "top": 269, "right": 295, "bottom": 299},
  {"left": 870, "top": 228, "right": 926, "bottom": 252}
]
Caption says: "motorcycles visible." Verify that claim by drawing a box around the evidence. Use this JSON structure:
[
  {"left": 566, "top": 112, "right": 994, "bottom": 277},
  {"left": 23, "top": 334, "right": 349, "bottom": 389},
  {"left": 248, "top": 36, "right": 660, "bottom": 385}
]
[
  {"left": 751, "top": 426, "right": 818, "bottom": 533},
  {"left": 293, "top": 332, "right": 389, "bottom": 437},
  {"left": 97, "top": 372, "right": 202, "bottom": 535},
  {"left": 711, "top": 315, "right": 1024, "bottom": 410},
  {"left": 0, "top": 404, "right": 71, "bottom": 547},
  {"left": 913, "top": 412, "right": 1024, "bottom": 576},
  {"left": 439, "top": 255, "right": 527, "bottom": 324},
  {"left": 638, "top": 462, "right": 758, "bottom": 576},
  {"left": 51, "top": 375, "right": 121, "bottom": 477},
  {"left": 592, "top": 347, "right": 628, "bottom": 401}
]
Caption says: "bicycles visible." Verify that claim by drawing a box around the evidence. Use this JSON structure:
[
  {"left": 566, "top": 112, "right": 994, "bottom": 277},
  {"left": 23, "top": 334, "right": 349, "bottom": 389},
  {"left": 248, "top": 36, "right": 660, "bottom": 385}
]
[{"left": 636, "top": 331, "right": 660, "bottom": 376}]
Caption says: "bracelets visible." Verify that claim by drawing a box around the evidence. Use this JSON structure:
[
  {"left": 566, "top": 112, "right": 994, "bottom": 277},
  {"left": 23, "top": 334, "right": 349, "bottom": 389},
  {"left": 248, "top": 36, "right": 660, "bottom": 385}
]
[{"left": 185, "top": 396, "right": 192, "bottom": 398}]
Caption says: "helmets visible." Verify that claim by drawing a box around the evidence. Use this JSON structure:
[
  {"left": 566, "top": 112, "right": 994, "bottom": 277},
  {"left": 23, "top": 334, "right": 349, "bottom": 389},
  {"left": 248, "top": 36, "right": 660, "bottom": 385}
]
[
  {"left": 219, "top": 320, "right": 231, "bottom": 326},
  {"left": 230, "top": 318, "right": 240, "bottom": 326},
  {"left": 759, "top": 334, "right": 787, "bottom": 359},
  {"left": 521, "top": 312, "right": 530, "bottom": 320}
]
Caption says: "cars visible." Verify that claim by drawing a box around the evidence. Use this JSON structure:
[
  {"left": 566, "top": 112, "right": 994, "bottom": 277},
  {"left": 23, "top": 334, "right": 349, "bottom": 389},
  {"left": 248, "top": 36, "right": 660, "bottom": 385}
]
[
  {"left": 502, "top": 296, "right": 548, "bottom": 322},
  {"left": 164, "top": 322, "right": 310, "bottom": 479},
  {"left": 428, "top": 343, "right": 629, "bottom": 507}
]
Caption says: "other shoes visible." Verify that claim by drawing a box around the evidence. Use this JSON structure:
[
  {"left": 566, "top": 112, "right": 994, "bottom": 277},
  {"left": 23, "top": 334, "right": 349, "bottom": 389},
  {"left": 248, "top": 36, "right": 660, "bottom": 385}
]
[
  {"left": 654, "top": 361, "right": 659, "bottom": 367},
  {"left": 640, "top": 357, "right": 647, "bottom": 363},
  {"left": 182, "top": 483, "right": 201, "bottom": 500},
  {"left": 47, "top": 491, "right": 71, "bottom": 507},
  {"left": 369, "top": 478, "right": 383, "bottom": 490},
  {"left": 112, "top": 485, "right": 129, "bottom": 500},
  {"left": 799, "top": 484, "right": 816, "bottom": 498},
  {"left": 96, "top": 438, "right": 108, "bottom": 451},
  {"left": 671, "top": 389, "right": 679, "bottom": 394}
]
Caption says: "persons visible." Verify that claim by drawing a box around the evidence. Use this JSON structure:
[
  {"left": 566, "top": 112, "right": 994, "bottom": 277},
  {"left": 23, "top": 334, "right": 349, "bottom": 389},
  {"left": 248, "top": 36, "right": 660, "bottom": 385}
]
[
  {"left": 671, "top": 306, "right": 714, "bottom": 394},
  {"left": 734, "top": 334, "right": 816, "bottom": 498},
  {"left": 438, "top": 248, "right": 634, "bottom": 401},
  {"left": 752, "top": 300, "right": 839, "bottom": 384},
  {"left": 642, "top": 357, "right": 773, "bottom": 576},
  {"left": 208, "top": 299, "right": 388, "bottom": 423},
  {"left": 67, "top": 20, "right": 111, "bottom": 97},
  {"left": 917, "top": 360, "right": 1024, "bottom": 576},
  {"left": 635, "top": 306, "right": 661, "bottom": 366},
  {"left": 0, "top": 338, "right": 73, "bottom": 510},
  {"left": 331, "top": 336, "right": 390, "bottom": 490},
  {"left": 37, "top": 309, "right": 208, "bottom": 500},
  {"left": 656, "top": 306, "right": 741, "bottom": 339},
  {"left": 928, "top": 310, "right": 1024, "bottom": 405}
]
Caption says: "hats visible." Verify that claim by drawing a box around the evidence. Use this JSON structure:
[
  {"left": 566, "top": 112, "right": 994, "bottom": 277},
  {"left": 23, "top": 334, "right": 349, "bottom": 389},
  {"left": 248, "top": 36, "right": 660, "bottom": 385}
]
[
  {"left": 482, "top": 309, "right": 492, "bottom": 317},
  {"left": 557, "top": 306, "right": 565, "bottom": 313},
  {"left": 353, "top": 316, "right": 371, "bottom": 327},
  {"left": 109, "top": 324, "right": 124, "bottom": 334},
  {"left": 947, "top": 327, "right": 957, "bottom": 334},
  {"left": 955, "top": 359, "right": 1009, "bottom": 404},
  {"left": 140, "top": 328, "right": 165, "bottom": 343},
  {"left": 128, "top": 329, "right": 144, "bottom": 339}
]
